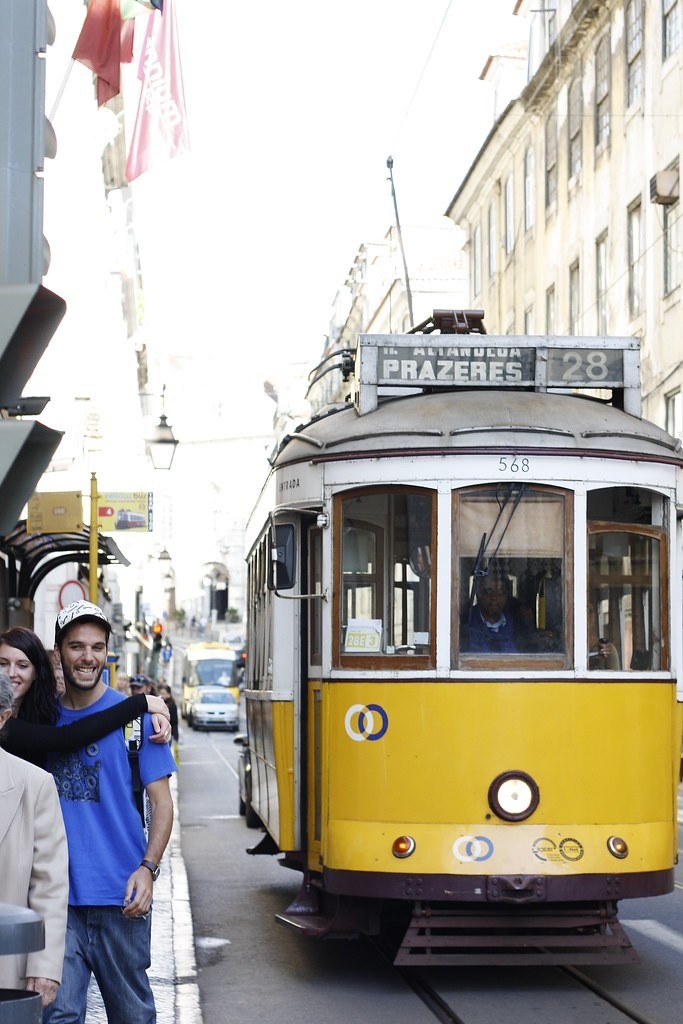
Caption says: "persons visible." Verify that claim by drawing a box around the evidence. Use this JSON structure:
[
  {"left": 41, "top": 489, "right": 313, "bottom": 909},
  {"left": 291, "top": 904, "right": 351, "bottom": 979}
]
[
  {"left": 0, "top": 600, "right": 179, "bottom": 1024},
  {"left": 0, "top": 669, "right": 69, "bottom": 1008},
  {"left": 217, "top": 670, "right": 231, "bottom": 686},
  {"left": 589, "top": 600, "right": 620, "bottom": 671},
  {"left": 458, "top": 566, "right": 555, "bottom": 654}
]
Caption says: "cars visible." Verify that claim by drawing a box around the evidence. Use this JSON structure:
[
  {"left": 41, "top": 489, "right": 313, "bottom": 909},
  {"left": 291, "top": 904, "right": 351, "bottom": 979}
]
[{"left": 185, "top": 686, "right": 240, "bottom": 733}]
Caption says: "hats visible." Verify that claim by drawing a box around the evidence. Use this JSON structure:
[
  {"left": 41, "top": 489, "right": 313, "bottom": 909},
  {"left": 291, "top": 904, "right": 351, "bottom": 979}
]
[
  {"left": 55, "top": 599, "right": 112, "bottom": 642},
  {"left": 129, "top": 674, "right": 149, "bottom": 687}
]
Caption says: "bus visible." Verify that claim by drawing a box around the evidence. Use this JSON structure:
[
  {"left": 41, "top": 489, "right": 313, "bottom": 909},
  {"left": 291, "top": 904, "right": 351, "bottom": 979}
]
[
  {"left": 114, "top": 509, "right": 146, "bottom": 529},
  {"left": 235, "top": 154, "right": 682, "bottom": 966},
  {"left": 181, "top": 641, "right": 239, "bottom": 719}
]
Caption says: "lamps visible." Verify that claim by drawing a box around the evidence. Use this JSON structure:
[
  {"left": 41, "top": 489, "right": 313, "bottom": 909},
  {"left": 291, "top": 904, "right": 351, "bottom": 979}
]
[{"left": 129, "top": 383, "right": 178, "bottom": 472}]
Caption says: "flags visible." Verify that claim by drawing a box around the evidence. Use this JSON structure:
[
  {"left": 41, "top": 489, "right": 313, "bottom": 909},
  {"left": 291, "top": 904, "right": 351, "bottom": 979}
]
[{"left": 71, "top": 0, "right": 190, "bottom": 181}]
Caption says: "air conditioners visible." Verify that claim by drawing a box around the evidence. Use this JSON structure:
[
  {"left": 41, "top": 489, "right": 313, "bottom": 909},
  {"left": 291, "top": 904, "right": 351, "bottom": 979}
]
[{"left": 648, "top": 170, "right": 679, "bottom": 204}]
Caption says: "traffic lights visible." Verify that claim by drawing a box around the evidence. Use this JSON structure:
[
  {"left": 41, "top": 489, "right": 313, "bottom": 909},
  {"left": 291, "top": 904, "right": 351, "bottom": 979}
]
[{"left": 152, "top": 622, "right": 163, "bottom": 652}]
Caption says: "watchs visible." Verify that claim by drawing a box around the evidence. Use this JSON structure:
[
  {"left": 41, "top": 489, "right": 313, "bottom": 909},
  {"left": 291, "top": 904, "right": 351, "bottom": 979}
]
[{"left": 141, "top": 860, "right": 161, "bottom": 881}]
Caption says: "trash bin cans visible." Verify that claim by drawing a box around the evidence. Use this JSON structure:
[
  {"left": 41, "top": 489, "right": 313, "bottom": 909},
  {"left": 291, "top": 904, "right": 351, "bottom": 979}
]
[{"left": 0, "top": 902, "right": 46, "bottom": 1024}]
